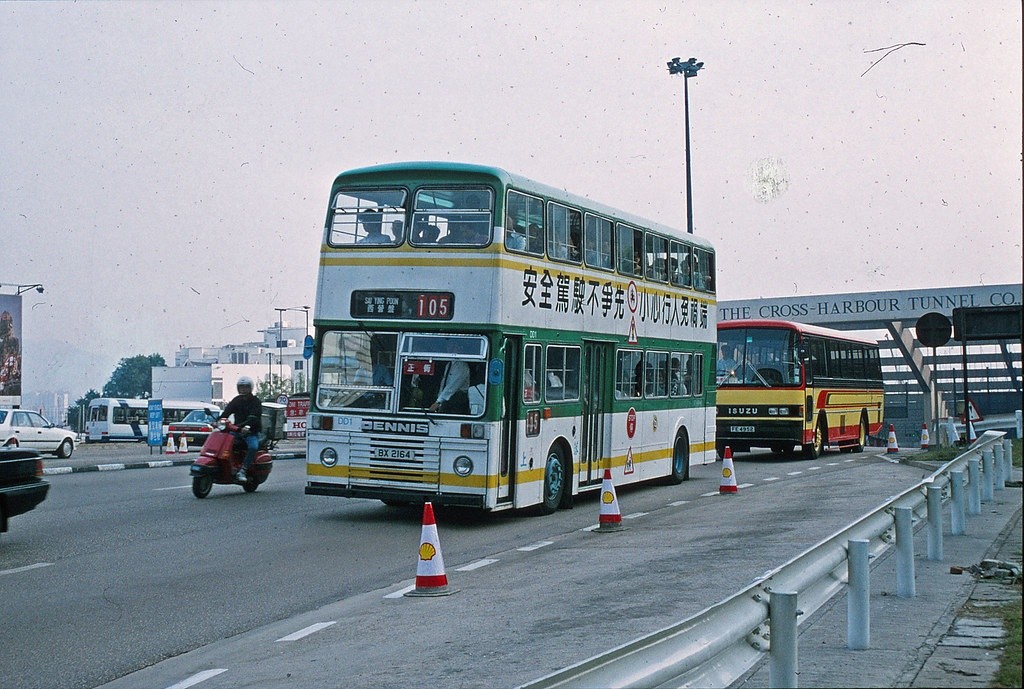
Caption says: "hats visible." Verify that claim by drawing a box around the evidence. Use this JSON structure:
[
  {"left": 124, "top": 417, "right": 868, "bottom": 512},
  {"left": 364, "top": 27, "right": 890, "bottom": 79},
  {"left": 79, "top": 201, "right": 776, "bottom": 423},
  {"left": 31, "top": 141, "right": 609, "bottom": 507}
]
[{"left": 357, "top": 209, "right": 380, "bottom": 221}]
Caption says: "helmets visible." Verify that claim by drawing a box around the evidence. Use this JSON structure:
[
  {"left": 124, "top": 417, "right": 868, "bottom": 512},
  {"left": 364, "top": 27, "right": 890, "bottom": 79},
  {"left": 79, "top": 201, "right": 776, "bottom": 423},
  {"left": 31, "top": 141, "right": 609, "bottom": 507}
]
[{"left": 236, "top": 377, "right": 254, "bottom": 395}]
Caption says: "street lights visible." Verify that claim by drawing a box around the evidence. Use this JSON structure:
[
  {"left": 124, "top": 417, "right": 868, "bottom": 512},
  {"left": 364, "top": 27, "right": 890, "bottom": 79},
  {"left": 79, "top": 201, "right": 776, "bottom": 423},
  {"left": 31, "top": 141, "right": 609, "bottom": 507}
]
[
  {"left": 266, "top": 305, "right": 312, "bottom": 400},
  {"left": 666, "top": 57, "right": 705, "bottom": 234}
]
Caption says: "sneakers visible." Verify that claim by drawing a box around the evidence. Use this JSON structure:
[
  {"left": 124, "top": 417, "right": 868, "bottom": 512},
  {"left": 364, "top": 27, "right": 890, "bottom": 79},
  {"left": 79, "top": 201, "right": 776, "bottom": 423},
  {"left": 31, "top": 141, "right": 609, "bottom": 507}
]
[{"left": 235, "top": 472, "right": 247, "bottom": 482}]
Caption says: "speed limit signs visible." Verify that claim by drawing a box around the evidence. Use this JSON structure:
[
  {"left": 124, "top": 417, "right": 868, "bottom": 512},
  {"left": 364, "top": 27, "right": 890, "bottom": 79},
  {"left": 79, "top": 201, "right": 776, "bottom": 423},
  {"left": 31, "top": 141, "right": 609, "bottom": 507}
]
[{"left": 276, "top": 395, "right": 290, "bottom": 405}]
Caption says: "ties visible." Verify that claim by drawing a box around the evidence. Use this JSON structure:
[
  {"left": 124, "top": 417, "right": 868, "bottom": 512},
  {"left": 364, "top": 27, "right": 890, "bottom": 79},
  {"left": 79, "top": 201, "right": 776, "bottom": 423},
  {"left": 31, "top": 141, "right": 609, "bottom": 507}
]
[{"left": 442, "top": 361, "right": 452, "bottom": 391}]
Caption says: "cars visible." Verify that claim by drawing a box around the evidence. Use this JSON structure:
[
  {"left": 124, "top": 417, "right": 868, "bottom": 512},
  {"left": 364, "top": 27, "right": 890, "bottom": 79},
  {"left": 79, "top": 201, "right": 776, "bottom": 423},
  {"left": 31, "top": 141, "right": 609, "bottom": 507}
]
[
  {"left": 0, "top": 447, "right": 50, "bottom": 532},
  {"left": 166, "top": 410, "right": 235, "bottom": 450},
  {"left": 0, "top": 409, "right": 79, "bottom": 459}
]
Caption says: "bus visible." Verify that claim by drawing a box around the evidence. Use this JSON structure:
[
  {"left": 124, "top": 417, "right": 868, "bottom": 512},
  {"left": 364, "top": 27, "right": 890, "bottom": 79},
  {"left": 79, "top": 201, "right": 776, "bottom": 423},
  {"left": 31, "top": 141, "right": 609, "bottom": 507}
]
[
  {"left": 85, "top": 397, "right": 222, "bottom": 444},
  {"left": 715, "top": 319, "right": 886, "bottom": 460},
  {"left": 302, "top": 160, "right": 718, "bottom": 516}
]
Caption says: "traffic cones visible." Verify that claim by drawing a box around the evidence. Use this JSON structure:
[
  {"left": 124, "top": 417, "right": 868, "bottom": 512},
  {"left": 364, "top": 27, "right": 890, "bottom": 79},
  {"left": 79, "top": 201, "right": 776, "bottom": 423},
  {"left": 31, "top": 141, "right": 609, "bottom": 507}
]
[
  {"left": 178, "top": 431, "right": 188, "bottom": 454},
  {"left": 969, "top": 421, "right": 978, "bottom": 444},
  {"left": 918, "top": 421, "right": 931, "bottom": 450},
  {"left": 713, "top": 446, "right": 743, "bottom": 496},
  {"left": 883, "top": 423, "right": 901, "bottom": 454},
  {"left": 165, "top": 433, "right": 177, "bottom": 454},
  {"left": 402, "top": 502, "right": 461, "bottom": 597},
  {"left": 590, "top": 468, "right": 631, "bottom": 532}
]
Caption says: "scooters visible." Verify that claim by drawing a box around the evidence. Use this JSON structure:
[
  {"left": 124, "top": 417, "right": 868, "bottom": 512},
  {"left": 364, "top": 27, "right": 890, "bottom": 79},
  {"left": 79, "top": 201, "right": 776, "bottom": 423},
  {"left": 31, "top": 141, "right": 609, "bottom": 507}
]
[{"left": 189, "top": 403, "right": 288, "bottom": 501}]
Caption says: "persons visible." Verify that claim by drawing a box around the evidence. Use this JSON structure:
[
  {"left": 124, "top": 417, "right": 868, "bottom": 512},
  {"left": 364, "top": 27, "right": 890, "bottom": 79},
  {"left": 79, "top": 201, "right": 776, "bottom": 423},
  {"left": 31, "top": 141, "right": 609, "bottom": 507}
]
[
  {"left": 507, "top": 216, "right": 526, "bottom": 251},
  {"left": 586, "top": 235, "right": 613, "bottom": 269},
  {"left": 716, "top": 345, "right": 738, "bottom": 376},
  {"left": 212, "top": 375, "right": 262, "bottom": 481},
  {"left": 429, "top": 339, "right": 471, "bottom": 416},
  {"left": 549, "top": 229, "right": 567, "bottom": 258},
  {"left": 357, "top": 209, "right": 391, "bottom": 244},
  {"left": 528, "top": 362, "right": 563, "bottom": 387},
  {"left": 411, "top": 212, "right": 440, "bottom": 244},
  {"left": 392, "top": 220, "right": 403, "bottom": 244},
  {"left": 570, "top": 232, "right": 581, "bottom": 262},
  {"left": 529, "top": 223, "right": 544, "bottom": 255},
  {"left": 647, "top": 254, "right": 716, "bottom": 290},
  {"left": 469, "top": 362, "right": 505, "bottom": 418},
  {"left": 616, "top": 356, "right": 693, "bottom": 398},
  {"left": 355, "top": 350, "right": 393, "bottom": 411},
  {"left": 619, "top": 246, "right": 642, "bottom": 276},
  {"left": 439, "top": 215, "right": 489, "bottom": 243}
]
